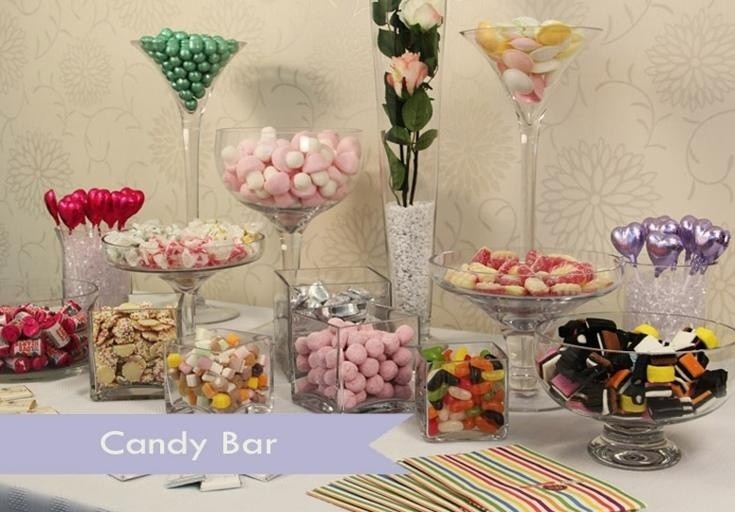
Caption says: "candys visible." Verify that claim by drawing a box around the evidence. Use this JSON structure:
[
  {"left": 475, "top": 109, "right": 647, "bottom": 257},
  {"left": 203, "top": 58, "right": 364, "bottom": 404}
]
[
  {"left": 533, "top": 317, "right": 729, "bottom": 426},
  {"left": 471, "top": 14, "right": 589, "bottom": 107},
  {"left": 421, "top": 345, "right": 507, "bottom": 438},
  {"left": 105, "top": 218, "right": 263, "bottom": 272},
  {"left": 441, "top": 245, "right": 616, "bottom": 299},
  {"left": 611, "top": 214, "right": 732, "bottom": 279},
  {"left": 138, "top": 25, "right": 241, "bottom": 114},
  {"left": 220, "top": 125, "right": 364, "bottom": 229},
  {"left": 91, "top": 299, "right": 272, "bottom": 415},
  {"left": 0, "top": 299, "right": 92, "bottom": 376},
  {"left": 42, "top": 185, "right": 146, "bottom": 231},
  {"left": 275, "top": 280, "right": 415, "bottom": 413}
]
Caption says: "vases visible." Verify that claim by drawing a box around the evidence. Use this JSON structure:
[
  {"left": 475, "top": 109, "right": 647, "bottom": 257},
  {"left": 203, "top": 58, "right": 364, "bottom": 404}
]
[
  {"left": 369, "top": 1, "right": 445, "bottom": 346},
  {"left": 611, "top": 250, "right": 721, "bottom": 346}
]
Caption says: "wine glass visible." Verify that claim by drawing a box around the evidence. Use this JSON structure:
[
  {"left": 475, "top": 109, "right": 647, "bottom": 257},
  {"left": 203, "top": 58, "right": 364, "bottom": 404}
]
[
  {"left": 457, "top": 25, "right": 604, "bottom": 354},
  {"left": 100, "top": 217, "right": 268, "bottom": 339},
  {"left": 531, "top": 312, "right": 735, "bottom": 472},
  {"left": 129, "top": 39, "right": 248, "bottom": 328},
  {"left": 430, "top": 248, "right": 622, "bottom": 411},
  {"left": 214, "top": 126, "right": 372, "bottom": 338}
]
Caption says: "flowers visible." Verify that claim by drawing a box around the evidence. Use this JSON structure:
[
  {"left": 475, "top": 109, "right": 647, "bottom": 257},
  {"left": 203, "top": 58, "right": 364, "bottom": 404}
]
[{"left": 370, "top": 1, "right": 446, "bottom": 208}]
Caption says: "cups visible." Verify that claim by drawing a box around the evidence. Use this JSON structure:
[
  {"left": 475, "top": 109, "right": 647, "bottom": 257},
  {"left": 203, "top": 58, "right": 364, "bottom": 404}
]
[
  {"left": 412, "top": 338, "right": 509, "bottom": 444},
  {"left": 163, "top": 328, "right": 273, "bottom": 418},
  {"left": 87, "top": 293, "right": 183, "bottom": 400},
  {"left": 270, "top": 264, "right": 392, "bottom": 385},
  {"left": 289, "top": 300, "right": 419, "bottom": 416},
  {"left": 621, "top": 255, "right": 707, "bottom": 339}
]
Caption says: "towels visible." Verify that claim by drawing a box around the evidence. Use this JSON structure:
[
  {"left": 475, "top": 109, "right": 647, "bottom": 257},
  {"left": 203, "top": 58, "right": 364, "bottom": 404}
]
[{"left": 305, "top": 443, "right": 646, "bottom": 512}]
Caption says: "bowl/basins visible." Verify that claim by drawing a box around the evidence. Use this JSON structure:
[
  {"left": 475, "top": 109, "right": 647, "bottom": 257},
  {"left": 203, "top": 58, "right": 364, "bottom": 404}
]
[{"left": 0, "top": 278, "right": 99, "bottom": 382}]
